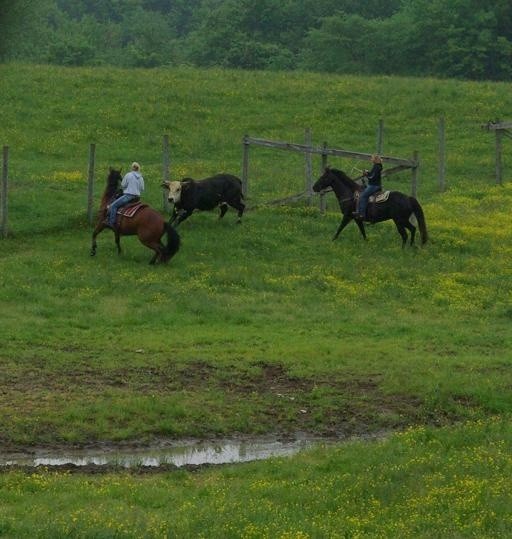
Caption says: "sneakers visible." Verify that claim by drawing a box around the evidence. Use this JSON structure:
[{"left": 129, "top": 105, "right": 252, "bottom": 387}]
[{"left": 351, "top": 211, "right": 365, "bottom": 222}]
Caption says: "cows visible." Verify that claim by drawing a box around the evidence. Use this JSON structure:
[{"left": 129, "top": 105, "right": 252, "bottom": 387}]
[{"left": 161, "top": 173, "right": 245, "bottom": 229}]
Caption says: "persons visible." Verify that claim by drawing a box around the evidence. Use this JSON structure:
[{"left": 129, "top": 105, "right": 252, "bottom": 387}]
[
  {"left": 106, "top": 161, "right": 144, "bottom": 225},
  {"left": 351, "top": 152, "right": 382, "bottom": 220}
]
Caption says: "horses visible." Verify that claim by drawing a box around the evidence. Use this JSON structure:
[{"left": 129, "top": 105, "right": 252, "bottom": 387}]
[
  {"left": 90, "top": 165, "right": 182, "bottom": 265},
  {"left": 312, "top": 167, "right": 428, "bottom": 250}
]
